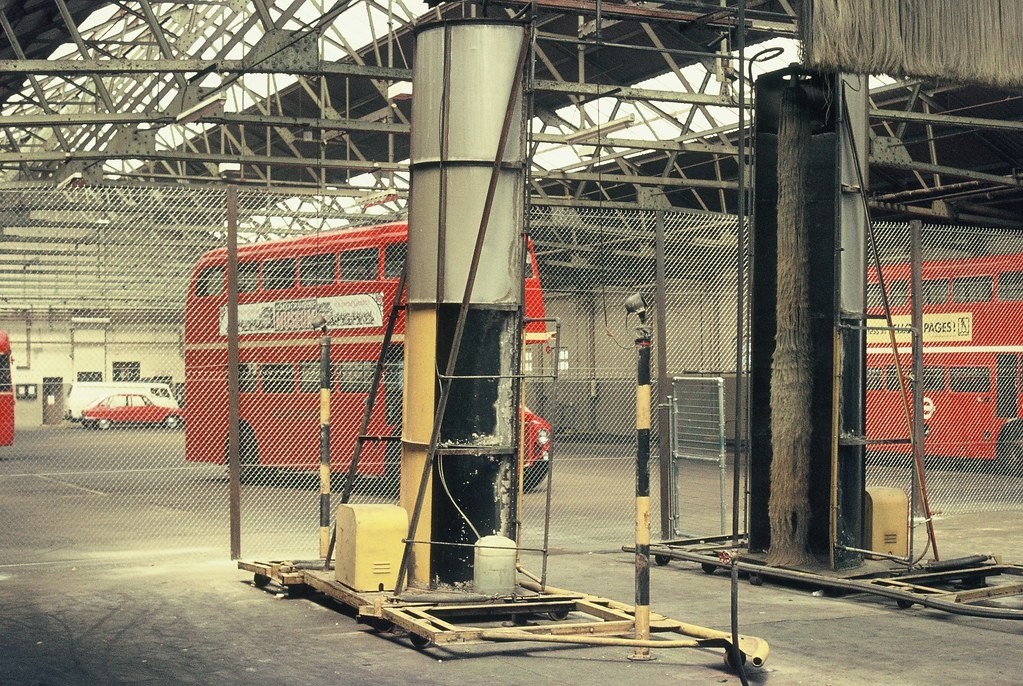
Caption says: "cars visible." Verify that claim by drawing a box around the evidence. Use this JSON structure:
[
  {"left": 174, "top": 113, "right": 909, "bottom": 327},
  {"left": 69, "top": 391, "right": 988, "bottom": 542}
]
[{"left": 81, "top": 393, "right": 183, "bottom": 430}]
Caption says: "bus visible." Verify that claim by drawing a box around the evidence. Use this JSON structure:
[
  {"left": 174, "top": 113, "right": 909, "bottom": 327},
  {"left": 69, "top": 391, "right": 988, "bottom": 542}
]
[
  {"left": 0, "top": 330, "right": 16, "bottom": 447},
  {"left": 183, "top": 221, "right": 552, "bottom": 497},
  {"left": 867, "top": 256, "right": 1022, "bottom": 479},
  {"left": 63, "top": 382, "right": 179, "bottom": 428}
]
